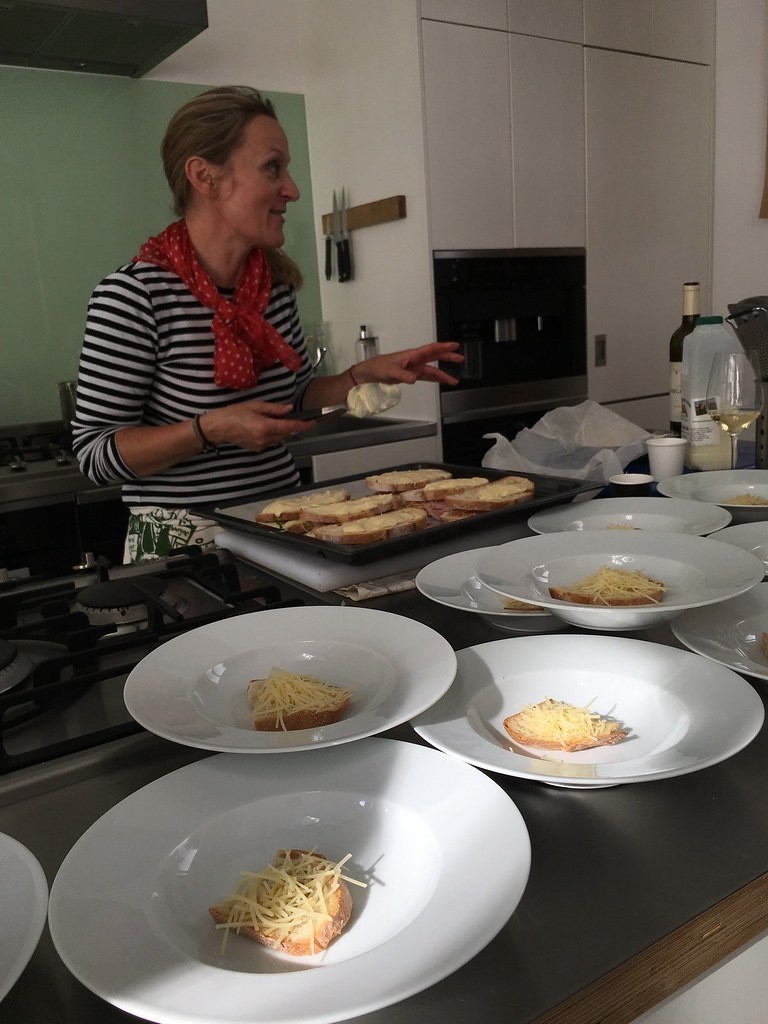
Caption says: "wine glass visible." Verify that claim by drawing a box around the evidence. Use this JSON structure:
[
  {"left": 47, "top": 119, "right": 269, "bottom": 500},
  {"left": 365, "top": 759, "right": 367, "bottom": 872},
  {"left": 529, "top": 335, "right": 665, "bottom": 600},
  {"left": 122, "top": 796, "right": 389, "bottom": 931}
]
[{"left": 705, "top": 350, "right": 764, "bottom": 469}]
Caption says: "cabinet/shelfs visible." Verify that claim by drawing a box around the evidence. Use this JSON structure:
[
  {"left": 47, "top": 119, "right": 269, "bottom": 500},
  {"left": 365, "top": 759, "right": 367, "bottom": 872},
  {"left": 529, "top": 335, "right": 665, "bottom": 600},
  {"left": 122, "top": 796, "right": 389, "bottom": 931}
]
[
  {"left": 584, "top": 0, "right": 717, "bottom": 65},
  {"left": 583, "top": 43, "right": 711, "bottom": 405},
  {"left": 421, "top": 0, "right": 584, "bottom": 46},
  {"left": 421, "top": 19, "right": 586, "bottom": 250}
]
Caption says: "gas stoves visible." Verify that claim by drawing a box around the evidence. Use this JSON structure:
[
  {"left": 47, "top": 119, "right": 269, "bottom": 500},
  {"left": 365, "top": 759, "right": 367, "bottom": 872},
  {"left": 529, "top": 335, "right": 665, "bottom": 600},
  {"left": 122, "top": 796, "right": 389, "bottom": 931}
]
[
  {"left": 0, "top": 421, "right": 87, "bottom": 485},
  {"left": 0, "top": 542, "right": 297, "bottom": 778}
]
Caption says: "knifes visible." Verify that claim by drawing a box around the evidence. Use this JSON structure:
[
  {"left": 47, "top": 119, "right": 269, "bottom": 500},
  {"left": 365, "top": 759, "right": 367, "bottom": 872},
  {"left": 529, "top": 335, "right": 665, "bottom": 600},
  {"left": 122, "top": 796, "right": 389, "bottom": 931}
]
[{"left": 325, "top": 187, "right": 352, "bottom": 283}]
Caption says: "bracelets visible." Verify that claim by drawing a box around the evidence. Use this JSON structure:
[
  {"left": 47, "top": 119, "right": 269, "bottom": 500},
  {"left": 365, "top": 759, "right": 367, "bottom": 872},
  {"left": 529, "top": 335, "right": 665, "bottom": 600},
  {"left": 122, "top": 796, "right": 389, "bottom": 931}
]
[
  {"left": 193, "top": 414, "right": 219, "bottom": 454},
  {"left": 348, "top": 367, "right": 358, "bottom": 386}
]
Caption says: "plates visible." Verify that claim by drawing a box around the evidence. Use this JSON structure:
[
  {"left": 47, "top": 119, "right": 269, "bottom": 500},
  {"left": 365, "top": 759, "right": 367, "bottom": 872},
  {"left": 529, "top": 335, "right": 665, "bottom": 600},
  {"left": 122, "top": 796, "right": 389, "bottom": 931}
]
[
  {"left": 124, "top": 603, "right": 460, "bottom": 753},
  {"left": 404, "top": 495, "right": 767, "bottom": 791},
  {"left": 0, "top": 833, "right": 49, "bottom": 1004},
  {"left": 47, "top": 737, "right": 532, "bottom": 1023},
  {"left": 657, "top": 470, "right": 768, "bottom": 522}
]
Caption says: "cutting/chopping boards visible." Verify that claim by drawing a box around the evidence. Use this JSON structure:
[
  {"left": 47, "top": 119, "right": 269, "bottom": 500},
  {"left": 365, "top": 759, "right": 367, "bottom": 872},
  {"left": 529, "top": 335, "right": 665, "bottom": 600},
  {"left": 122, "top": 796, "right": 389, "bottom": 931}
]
[{"left": 214, "top": 521, "right": 532, "bottom": 593}]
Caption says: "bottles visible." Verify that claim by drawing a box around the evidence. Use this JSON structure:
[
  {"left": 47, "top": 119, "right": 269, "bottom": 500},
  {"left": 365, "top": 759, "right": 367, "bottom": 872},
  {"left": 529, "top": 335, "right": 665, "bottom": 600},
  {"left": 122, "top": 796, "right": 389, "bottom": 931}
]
[
  {"left": 680, "top": 316, "right": 741, "bottom": 471},
  {"left": 670, "top": 282, "right": 704, "bottom": 435}
]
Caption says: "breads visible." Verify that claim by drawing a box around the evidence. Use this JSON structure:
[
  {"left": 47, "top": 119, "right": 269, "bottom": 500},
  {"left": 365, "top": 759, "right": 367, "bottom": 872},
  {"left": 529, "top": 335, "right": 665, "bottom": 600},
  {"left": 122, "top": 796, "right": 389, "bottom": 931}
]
[
  {"left": 255, "top": 466, "right": 536, "bottom": 546},
  {"left": 208, "top": 849, "right": 354, "bottom": 955},
  {"left": 246, "top": 678, "right": 354, "bottom": 731},
  {"left": 504, "top": 570, "right": 662, "bottom": 611},
  {"left": 502, "top": 699, "right": 631, "bottom": 752}
]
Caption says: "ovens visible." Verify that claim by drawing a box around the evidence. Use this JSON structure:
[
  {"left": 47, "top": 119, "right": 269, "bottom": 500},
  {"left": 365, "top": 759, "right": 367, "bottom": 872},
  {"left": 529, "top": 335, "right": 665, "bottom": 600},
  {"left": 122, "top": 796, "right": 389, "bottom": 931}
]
[{"left": 429, "top": 245, "right": 591, "bottom": 473}]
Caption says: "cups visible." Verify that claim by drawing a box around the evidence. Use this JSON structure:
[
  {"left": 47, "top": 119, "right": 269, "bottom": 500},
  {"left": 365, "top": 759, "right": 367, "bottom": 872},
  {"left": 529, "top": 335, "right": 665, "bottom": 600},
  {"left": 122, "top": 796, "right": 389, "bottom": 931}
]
[
  {"left": 646, "top": 437, "right": 688, "bottom": 484},
  {"left": 608, "top": 474, "right": 654, "bottom": 496}
]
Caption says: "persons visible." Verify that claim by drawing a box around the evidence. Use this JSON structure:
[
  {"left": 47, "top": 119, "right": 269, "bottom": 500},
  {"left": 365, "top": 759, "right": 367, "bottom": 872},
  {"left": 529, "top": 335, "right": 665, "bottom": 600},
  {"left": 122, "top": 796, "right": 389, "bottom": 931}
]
[{"left": 72, "top": 87, "right": 464, "bottom": 564}]
